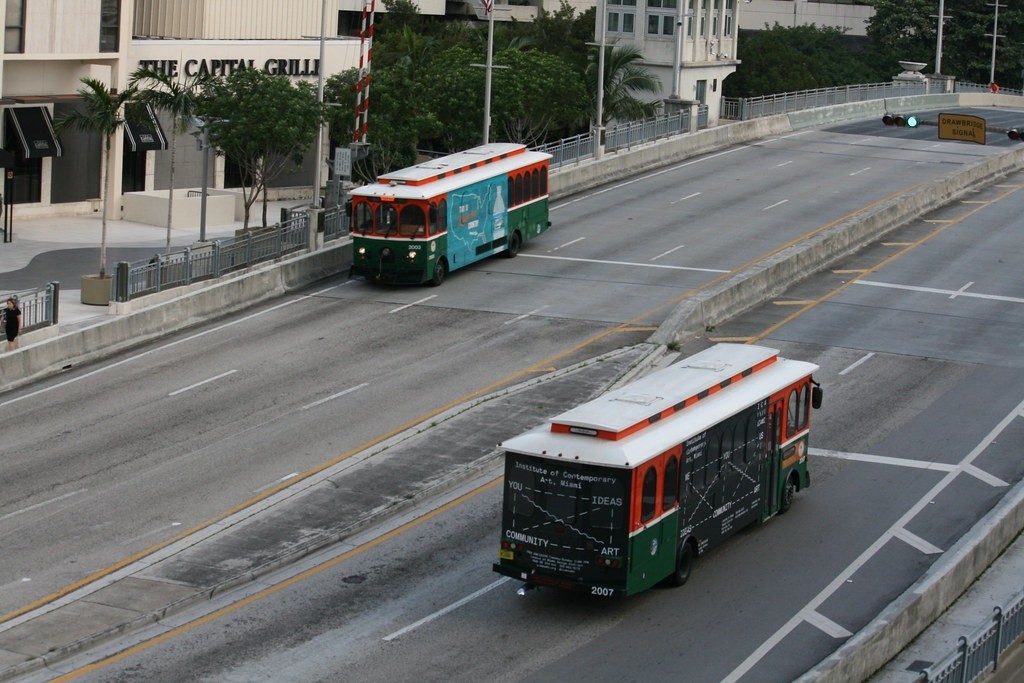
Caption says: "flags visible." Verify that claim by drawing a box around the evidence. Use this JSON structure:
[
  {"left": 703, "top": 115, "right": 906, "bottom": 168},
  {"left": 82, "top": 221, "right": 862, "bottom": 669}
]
[{"left": 479, "top": 0, "right": 492, "bottom": 15}]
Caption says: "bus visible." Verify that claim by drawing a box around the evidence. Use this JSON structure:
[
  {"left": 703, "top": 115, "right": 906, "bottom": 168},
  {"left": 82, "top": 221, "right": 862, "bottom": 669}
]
[
  {"left": 495, "top": 338, "right": 825, "bottom": 602},
  {"left": 345, "top": 141, "right": 555, "bottom": 289}
]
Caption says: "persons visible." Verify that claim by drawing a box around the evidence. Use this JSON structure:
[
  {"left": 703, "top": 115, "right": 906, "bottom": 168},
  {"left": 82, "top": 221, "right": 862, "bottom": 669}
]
[
  {"left": 987, "top": 81, "right": 1000, "bottom": 93},
  {"left": 0, "top": 298, "right": 23, "bottom": 350}
]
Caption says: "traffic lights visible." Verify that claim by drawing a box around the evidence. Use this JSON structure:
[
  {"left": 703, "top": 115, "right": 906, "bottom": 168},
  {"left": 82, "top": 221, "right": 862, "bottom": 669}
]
[
  {"left": 1007, "top": 127, "right": 1024, "bottom": 142},
  {"left": 881, "top": 112, "right": 920, "bottom": 129}
]
